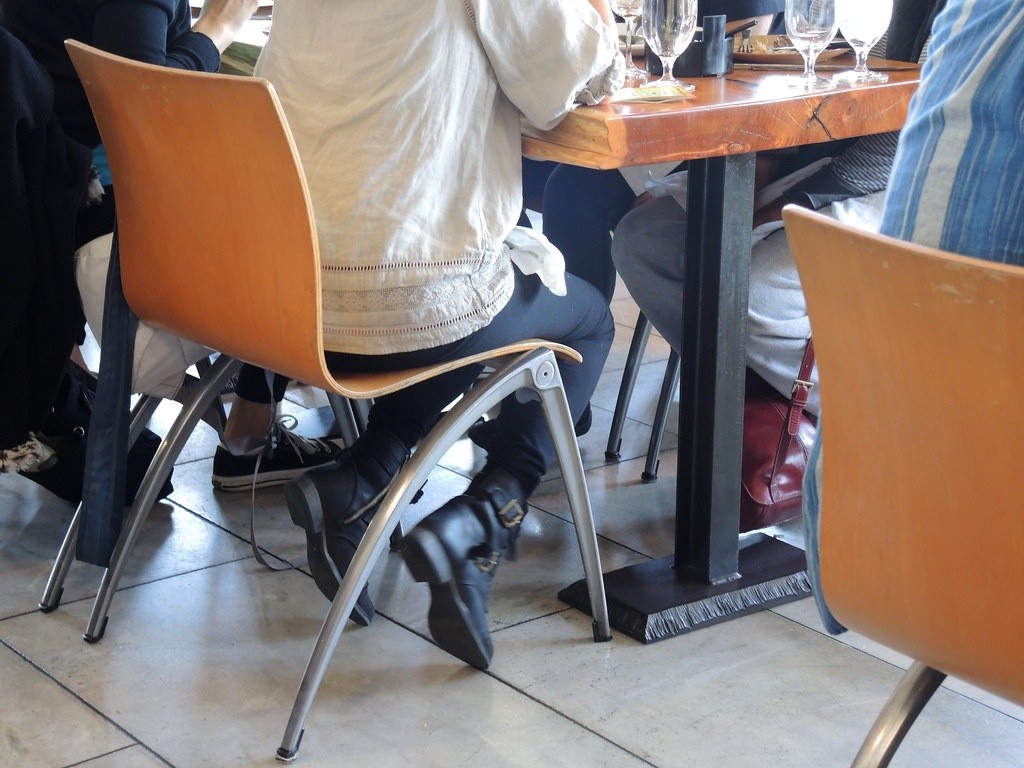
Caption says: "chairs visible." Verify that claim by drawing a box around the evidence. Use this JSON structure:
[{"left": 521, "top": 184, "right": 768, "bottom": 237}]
[
  {"left": 60, "top": 39, "right": 611, "bottom": 761},
  {"left": 779, "top": 203, "right": 1024, "bottom": 768}
]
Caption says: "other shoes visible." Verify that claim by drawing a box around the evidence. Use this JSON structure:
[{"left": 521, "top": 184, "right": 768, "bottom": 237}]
[{"left": 575, "top": 402, "right": 593, "bottom": 436}]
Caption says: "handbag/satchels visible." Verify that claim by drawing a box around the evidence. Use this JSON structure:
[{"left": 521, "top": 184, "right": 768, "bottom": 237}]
[
  {"left": 739, "top": 394, "right": 817, "bottom": 533},
  {"left": 0, "top": 347, "right": 174, "bottom": 505}
]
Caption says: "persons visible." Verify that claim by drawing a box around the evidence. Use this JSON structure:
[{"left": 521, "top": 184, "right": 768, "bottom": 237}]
[
  {"left": 468, "top": 0, "right": 788, "bottom": 450},
  {"left": 803, "top": 0, "right": 1024, "bottom": 635},
  {"left": 0, "top": 0, "right": 346, "bottom": 489},
  {"left": 614, "top": 0, "right": 948, "bottom": 417},
  {"left": 255, "top": 0, "right": 627, "bottom": 670}
]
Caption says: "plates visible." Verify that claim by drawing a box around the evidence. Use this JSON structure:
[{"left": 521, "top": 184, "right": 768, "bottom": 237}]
[
  {"left": 616, "top": 23, "right": 646, "bottom": 57},
  {"left": 731, "top": 35, "right": 850, "bottom": 64}
]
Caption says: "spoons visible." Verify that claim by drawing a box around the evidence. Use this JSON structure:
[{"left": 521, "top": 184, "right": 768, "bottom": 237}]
[{"left": 618, "top": 15, "right": 644, "bottom": 43}]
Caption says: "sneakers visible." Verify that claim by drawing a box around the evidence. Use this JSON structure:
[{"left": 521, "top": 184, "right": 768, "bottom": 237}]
[{"left": 212, "top": 412, "right": 346, "bottom": 570}]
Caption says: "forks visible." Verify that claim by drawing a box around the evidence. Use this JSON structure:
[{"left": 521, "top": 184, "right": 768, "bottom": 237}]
[{"left": 738, "top": 30, "right": 753, "bottom": 53}]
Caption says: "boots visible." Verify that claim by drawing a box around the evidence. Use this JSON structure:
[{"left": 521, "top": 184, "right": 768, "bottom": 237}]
[
  {"left": 402, "top": 456, "right": 528, "bottom": 670},
  {"left": 284, "top": 425, "right": 428, "bottom": 626}
]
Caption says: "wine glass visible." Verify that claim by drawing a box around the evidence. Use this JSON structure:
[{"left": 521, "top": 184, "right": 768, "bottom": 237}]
[
  {"left": 784, "top": 0, "right": 838, "bottom": 89},
  {"left": 640, "top": 0, "right": 698, "bottom": 91},
  {"left": 608, "top": 0, "right": 651, "bottom": 80},
  {"left": 835, "top": 0, "right": 893, "bottom": 85}
]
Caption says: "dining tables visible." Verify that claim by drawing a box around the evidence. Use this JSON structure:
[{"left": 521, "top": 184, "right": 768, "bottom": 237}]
[{"left": 517, "top": 52, "right": 926, "bottom": 645}]
